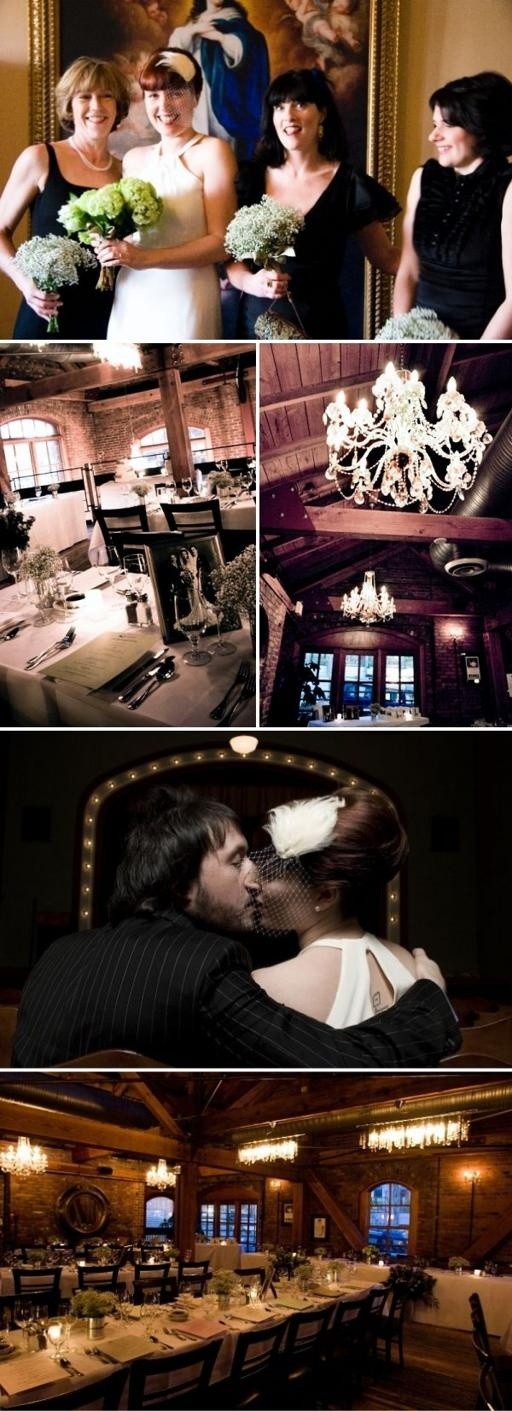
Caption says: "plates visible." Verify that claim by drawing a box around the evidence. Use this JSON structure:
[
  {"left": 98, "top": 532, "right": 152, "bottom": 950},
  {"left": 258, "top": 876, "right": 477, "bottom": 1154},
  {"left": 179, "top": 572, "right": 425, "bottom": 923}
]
[{"left": 168, "top": 1309, "right": 189, "bottom": 1321}]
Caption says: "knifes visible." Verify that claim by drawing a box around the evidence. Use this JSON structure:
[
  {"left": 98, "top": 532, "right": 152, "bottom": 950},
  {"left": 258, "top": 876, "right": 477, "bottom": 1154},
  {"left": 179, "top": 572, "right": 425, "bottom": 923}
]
[
  {"left": 117, "top": 657, "right": 176, "bottom": 703},
  {"left": 112, "top": 646, "right": 169, "bottom": 695}
]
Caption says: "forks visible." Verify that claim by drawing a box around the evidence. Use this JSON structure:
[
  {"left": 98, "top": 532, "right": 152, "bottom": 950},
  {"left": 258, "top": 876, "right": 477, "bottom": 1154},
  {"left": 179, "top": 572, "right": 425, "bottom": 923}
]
[
  {"left": 209, "top": 661, "right": 250, "bottom": 721},
  {"left": 219, "top": 676, "right": 254, "bottom": 726},
  {"left": 84, "top": 1347, "right": 118, "bottom": 1365},
  {"left": 26, "top": 626, "right": 75, "bottom": 671}
]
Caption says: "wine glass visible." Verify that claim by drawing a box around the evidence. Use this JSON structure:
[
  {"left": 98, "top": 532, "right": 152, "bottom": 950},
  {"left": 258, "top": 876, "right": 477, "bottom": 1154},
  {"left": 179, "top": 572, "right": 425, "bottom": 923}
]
[
  {"left": 1, "top": 546, "right": 29, "bottom": 603},
  {"left": 0, "top": 1310, "right": 10, "bottom": 1347},
  {"left": 173, "top": 587, "right": 215, "bottom": 667},
  {"left": 116, "top": 1287, "right": 161, "bottom": 1343},
  {"left": 97, "top": 547, "right": 124, "bottom": 600},
  {"left": 197, "top": 565, "right": 242, "bottom": 658},
  {"left": 14, "top": 1301, "right": 32, "bottom": 1349},
  {"left": 241, "top": 471, "right": 251, "bottom": 498},
  {"left": 32, "top": 1305, "right": 48, "bottom": 1349},
  {"left": 52, "top": 556, "right": 75, "bottom": 624},
  {"left": 182, "top": 478, "right": 194, "bottom": 499},
  {"left": 23, "top": 575, "right": 56, "bottom": 628},
  {"left": 123, "top": 553, "right": 150, "bottom": 614},
  {"left": 165, "top": 480, "right": 179, "bottom": 503},
  {"left": 47, "top": 1319, "right": 68, "bottom": 1361}
]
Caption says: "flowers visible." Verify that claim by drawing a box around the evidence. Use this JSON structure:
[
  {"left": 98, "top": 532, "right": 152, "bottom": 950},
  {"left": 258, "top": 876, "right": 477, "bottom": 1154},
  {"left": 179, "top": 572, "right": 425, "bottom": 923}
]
[
  {"left": 370, "top": 303, "right": 464, "bottom": 341},
  {"left": 53, "top": 175, "right": 165, "bottom": 293},
  {"left": 13, "top": 231, "right": 101, "bottom": 334},
  {"left": 223, "top": 193, "right": 308, "bottom": 277}
]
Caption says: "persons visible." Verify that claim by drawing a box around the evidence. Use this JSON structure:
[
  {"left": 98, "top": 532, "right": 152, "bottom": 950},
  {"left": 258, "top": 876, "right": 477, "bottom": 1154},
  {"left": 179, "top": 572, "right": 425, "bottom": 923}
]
[
  {"left": 395, "top": 71, "right": 512, "bottom": 339},
  {"left": 107, "top": 0, "right": 366, "bottom": 167},
  {"left": 244, "top": 786, "right": 416, "bottom": 1029},
  {"left": 285, "top": 1206, "right": 293, "bottom": 1220},
  {"left": 225, "top": 68, "right": 402, "bottom": 340},
  {"left": 94, "top": 49, "right": 237, "bottom": 339},
  {"left": 0, "top": 56, "right": 122, "bottom": 340},
  {"left": 9, "top": 787, "right": 463, "bottom": 1067}
]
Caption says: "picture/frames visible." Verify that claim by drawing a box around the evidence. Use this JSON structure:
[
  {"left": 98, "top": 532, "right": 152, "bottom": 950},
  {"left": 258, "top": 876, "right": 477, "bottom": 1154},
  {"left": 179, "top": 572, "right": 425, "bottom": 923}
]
[
  {"left": 280, "top": 1199, "right": 293, "bottom": 1227},
  {"left": 308, "top": 1213, "right": 331, "bottom": 1244},
  {"left": 27, "top": 0, "right": 402, "bottom": 345}
]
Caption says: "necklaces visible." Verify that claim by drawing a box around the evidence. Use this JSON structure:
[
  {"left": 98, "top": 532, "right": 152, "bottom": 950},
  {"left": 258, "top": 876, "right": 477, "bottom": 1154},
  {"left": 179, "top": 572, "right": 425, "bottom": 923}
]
[{"left": 67, "top": 134, "right": 113, "bottom": 171}]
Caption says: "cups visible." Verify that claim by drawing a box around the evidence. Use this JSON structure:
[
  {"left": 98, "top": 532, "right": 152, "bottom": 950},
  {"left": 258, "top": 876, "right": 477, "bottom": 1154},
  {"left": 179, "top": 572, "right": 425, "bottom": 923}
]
[
  {"left": 215, "top": 454, "right": 230, "bottom": 473},
  {"left": 157, "top": 487, "right": 165, "bottom": 501},
  {"left": 57, "top": 1304, "right": 78, "bottom": 1328}
]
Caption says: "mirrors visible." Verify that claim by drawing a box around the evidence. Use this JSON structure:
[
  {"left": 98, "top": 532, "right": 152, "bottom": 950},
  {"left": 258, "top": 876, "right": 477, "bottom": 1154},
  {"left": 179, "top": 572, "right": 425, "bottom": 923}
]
[{"left": 54, "top": 1180, "right": 112, "bottom": 1244}]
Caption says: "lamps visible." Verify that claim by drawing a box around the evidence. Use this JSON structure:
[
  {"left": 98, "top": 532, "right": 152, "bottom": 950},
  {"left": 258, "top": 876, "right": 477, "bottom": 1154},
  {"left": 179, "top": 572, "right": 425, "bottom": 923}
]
[
  {"left": 341, "top": 542, "right": 399, "bottom": 626},
  {"left": 352, "top": 1108, "right": 475, "bottom": 1153},
  {"left": 228, "top": 733, "right": 261, "bottom": 758},
  {"left": 2, "top": 1130, "right": 50, "bottom": 1182},
  {"left": 143, "top": 1153, "right": 179, "bottom": 1194},
  {"left": 319, "top": 345, "right": 494, "bottom": 514},
  {"left": 235, "top": 1133, "right": 306, "bottom": 1167}
]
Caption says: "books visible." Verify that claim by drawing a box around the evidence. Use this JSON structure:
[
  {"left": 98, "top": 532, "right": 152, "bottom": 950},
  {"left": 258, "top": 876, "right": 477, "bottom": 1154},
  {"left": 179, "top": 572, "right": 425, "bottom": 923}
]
[
  {"left": 277, "top": 1295, "right": 313, "bottom": 1311},
  {"left": 231, "top": 1305, "right": 279, "bottom": 1324},
  {"left": 1, "top": 1353, "right": 71, "bottom": 1397},
  {"left": 94, "top": 1334, "right": 157, "bottom": 1367},
  {"left": 174, "top": 1316, "right": 227, "bottom": 1340},
  {"left": 309, "top": 1286, "right": 347, "bottom": 1299},
  {"left": 342, "top": 1278, "right": 374, "bottom": 1290}
]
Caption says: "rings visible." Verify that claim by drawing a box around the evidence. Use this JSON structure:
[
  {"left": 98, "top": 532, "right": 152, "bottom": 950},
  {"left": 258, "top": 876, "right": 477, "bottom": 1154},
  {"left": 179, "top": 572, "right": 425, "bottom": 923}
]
[
  {"left": 114, "top": 249, "right": 123, "bottom": 259},
  {"left": 265, "top": 280, "right": 274, "bottom": 289}
]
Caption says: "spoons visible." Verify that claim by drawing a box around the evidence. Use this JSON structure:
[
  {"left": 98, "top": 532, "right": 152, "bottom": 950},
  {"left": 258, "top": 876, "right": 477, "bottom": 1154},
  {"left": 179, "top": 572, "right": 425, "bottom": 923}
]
[
  {"left": 127, "top": 663, "right": 175, "bottom": 710},
  {"left": 60, "top": 1358, "right": 84, "bottom": 1379},
  {"left": 0, "top": 628, "right": 21, "bottom": 642}
]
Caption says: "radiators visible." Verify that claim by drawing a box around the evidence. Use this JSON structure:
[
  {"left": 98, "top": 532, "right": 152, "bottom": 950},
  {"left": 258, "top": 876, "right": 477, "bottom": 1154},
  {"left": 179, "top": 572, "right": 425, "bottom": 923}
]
[{"left": 2, "top": 469, "right": 255, "bottom": 727}]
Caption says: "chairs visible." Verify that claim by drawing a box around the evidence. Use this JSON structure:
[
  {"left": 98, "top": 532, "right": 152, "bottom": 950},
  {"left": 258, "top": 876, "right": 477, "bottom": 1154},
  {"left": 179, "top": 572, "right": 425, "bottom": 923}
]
[
  {"left": 0, "top": 1007, "right": 21, "bottom": 1066},
  {"left": 49, "top": 1046, "right": 175, "bottom": 1067},
  {"left": 439, "top": 1052, "right": 512, "bottom": 1068}
]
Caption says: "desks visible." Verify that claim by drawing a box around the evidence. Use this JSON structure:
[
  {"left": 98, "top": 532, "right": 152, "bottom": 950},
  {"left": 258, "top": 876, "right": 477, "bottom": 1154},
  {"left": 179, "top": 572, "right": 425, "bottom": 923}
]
[{"left": 308, "top": 703, "right": 435, "bottom": 726}]
